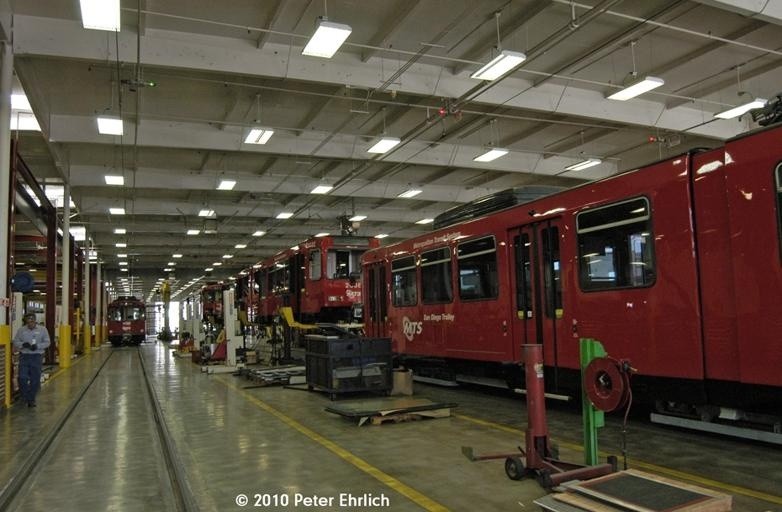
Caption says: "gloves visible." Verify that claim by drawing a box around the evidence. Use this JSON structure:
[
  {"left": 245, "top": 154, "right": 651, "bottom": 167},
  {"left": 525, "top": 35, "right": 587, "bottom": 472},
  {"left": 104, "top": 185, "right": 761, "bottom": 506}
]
[{"left": 23, "top": 342, "right": 37, "bottom": 352}]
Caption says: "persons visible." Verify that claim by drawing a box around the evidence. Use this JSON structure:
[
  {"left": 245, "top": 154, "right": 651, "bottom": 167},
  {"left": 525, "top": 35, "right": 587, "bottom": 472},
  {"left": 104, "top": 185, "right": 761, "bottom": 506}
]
[{"left": 12, "top": 313, "right": 52, "bottom": 407}]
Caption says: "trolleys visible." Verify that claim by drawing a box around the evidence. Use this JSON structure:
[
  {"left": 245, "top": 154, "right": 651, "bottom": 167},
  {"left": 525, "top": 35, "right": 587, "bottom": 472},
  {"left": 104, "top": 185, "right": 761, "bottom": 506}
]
[
  {"left": 505, "top": 344, "right": 617, "bottom": 490},
  {"left": 303, "top": 331, "right": 393, "bottom": 401}
]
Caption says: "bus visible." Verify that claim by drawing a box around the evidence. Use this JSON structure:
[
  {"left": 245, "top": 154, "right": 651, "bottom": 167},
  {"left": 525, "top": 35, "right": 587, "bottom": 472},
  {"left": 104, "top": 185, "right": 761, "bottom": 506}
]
[
  {"left": 201, "top": 281, "right": 232, "bottom": 325},
  {"left": 335, "top": 120, "right": 782, "bottom": 434},
  {"left": 237, "top": 233, "right": 380, "bottom": 332},
  {"left": 105, "top": 295, "right": 146, "bottom": 347}
]
